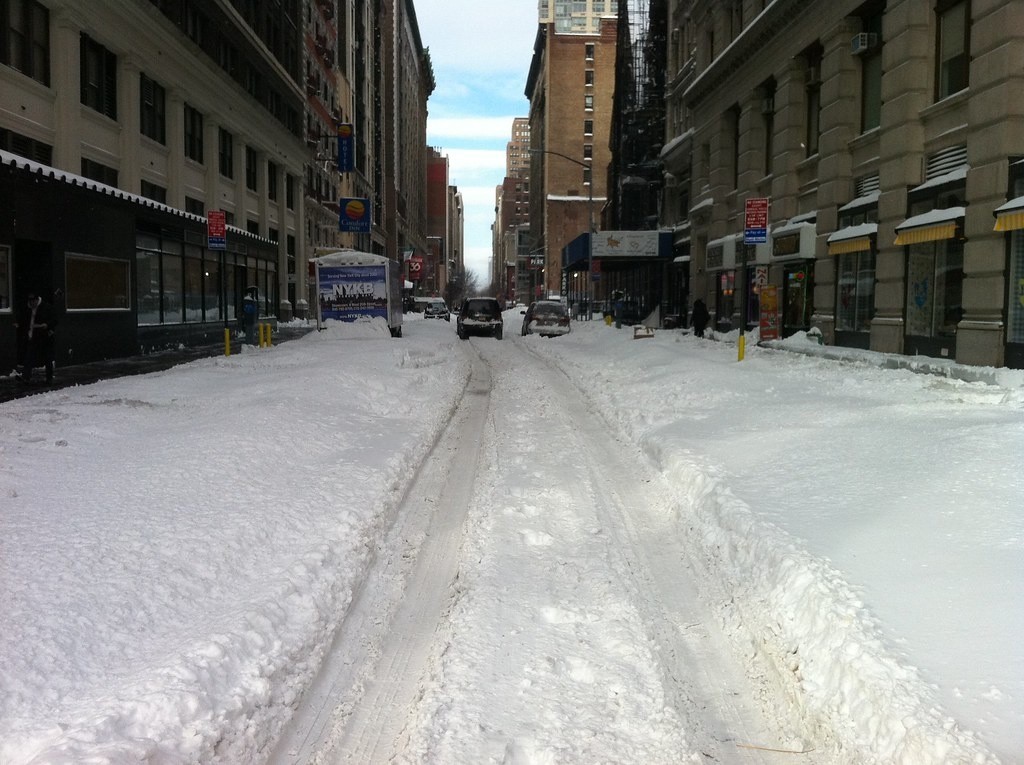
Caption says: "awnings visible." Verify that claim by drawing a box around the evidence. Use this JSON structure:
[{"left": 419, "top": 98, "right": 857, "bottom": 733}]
[
  {"left": 893, "top": 216, "right": 965, "bottom": 245},
  {"left": 992, "top": 206, "right": 1024, "bottom": 231},
  {"left": 827, "top": 232, "right": 877, "bottom": 255}
]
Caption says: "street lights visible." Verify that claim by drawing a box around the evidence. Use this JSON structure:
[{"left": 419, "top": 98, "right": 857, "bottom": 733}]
[{"left": 527, "top": 149, "right": 593, "bottom": 321}]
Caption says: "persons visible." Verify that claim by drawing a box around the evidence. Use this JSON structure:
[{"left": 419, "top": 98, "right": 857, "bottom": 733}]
[
  {"left": 15, "top": 293, "right": 59, "bottom": 387},
  {"left": 242, "top": 296, "right": 257, "bottom": 344},
  {"left": 690, "top": 299, "right": 708, "bottom": 338}
]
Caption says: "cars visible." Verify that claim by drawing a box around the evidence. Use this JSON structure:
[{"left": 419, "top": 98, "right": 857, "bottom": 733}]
[
  {"left": 454, "top": 296, "right": 503, "bottom": 341},
  {"left": 424, "top": 301, "right": 450, "bottom": 322},
  {"left": 520, "top": 301, "right": 571, "bottom": 337}
]
